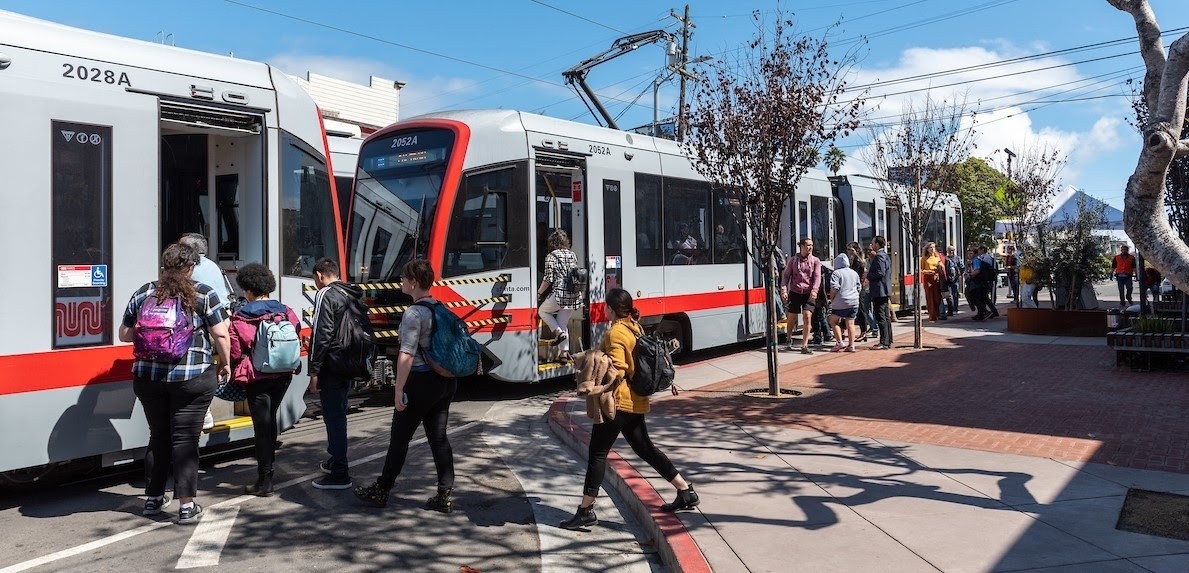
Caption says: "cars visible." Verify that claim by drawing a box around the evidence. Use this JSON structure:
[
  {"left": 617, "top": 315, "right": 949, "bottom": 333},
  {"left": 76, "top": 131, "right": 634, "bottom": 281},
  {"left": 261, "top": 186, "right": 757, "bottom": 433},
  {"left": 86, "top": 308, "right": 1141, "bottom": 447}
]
[{"left": 998, "top": 267, "right": 1007, "bottom": 286}]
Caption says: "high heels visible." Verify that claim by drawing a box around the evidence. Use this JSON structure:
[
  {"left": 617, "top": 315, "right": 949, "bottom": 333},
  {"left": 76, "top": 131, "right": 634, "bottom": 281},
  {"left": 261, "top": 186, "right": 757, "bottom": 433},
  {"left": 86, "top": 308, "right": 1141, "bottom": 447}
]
[{"left": 854, "top": 332, "right": 868, "bottom": 342}]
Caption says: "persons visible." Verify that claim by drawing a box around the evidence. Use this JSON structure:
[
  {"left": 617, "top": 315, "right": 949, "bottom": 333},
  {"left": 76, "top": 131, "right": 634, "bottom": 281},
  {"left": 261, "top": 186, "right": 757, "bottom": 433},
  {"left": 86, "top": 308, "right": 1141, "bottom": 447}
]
[
  {"left": 861, "top": 236, "right": 894, "bottom": 350},
  {"left": 308, "top": 257, "right": 362, "bottom": 488},
  {"left": 1143, "top": 267, "right": 1161, "bottom": 313},
  {"left": 536, "top": 228, "right": 584, "bottom": 364},
  {"left": 828, "top": 252, "right": 862, "bottom": 354},
  {"left": 228, "top": 262, "right": 301, "bottom": 498},
  {"left": 847, "top": 241, "right": 897, "bottom": 343},
  {"left": 779, "top": 238, "right": 822, "bottom": 354},
  {"left": 764, "top": 240, "right": 788, "bottom": 322},
  {"left": 557, "top": 288, "right": 701, "bottom": 530},
  {"left": 350, "top": 258, "right": 458, "bottom": 514},
  {"left": 1109, "top": 246, "right": 1137, "bottom": 309},
  {"left": 631, "top": 214, "right": 740, "bottom": 265},
  {"left": 119, "top": 244, "right": 232, "bottom": 525},
  {"left": 177, "top": 233, "right": 237, "bottom": 431},
  {"left": 920, "top": 238, "right": 1047, "bottom": 323},
  {"left": 808, "top": 251, "right": 835, "bottom": 345}
]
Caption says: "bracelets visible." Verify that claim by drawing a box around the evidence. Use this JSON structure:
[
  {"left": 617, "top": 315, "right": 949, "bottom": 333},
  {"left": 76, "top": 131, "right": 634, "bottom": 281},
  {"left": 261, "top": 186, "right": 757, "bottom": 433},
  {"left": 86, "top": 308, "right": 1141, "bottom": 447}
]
[{"left": 537, "top": 292, "right": 542, "bottom": 296}]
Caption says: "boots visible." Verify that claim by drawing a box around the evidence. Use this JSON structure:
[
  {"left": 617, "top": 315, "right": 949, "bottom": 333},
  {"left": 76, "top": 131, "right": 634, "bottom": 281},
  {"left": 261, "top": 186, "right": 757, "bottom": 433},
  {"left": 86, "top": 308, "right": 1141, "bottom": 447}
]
[
  {"left": 559, "top": 504, "right": 597, "bottom": 530},
  {"left": 989, "top": 305, "right": 1000, "bottom": 316},
  {"left": 660, "top": 482, "right": 700, "bottom": 511},
  {"left": 426, "top": 488, "right": 452, "bottom": 512},
  {"left": 352, "top": 478, "right": 389, "bottom": 507}
]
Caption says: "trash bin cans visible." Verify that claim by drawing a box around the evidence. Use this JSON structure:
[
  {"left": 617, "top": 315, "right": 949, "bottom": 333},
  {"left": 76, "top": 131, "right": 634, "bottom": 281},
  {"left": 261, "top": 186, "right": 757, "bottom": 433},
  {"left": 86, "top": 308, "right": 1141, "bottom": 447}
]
[{"left": 1001, "top": 276, "right": 1008, "bottom": 286}]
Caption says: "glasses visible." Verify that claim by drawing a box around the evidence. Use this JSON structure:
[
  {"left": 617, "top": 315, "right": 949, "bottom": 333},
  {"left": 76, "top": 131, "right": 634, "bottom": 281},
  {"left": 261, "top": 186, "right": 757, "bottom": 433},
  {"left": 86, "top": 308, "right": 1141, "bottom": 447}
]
[{"left": 400, "top": 275, "right": 409, "bottom": 281}]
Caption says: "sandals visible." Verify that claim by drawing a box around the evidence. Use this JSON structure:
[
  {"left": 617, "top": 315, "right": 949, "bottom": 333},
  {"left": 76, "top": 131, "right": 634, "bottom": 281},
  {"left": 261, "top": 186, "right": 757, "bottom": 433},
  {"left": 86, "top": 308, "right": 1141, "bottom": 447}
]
[
  {"left": 553, "top": 354, "right": 568, "bottom": 364},
  {"left": 549, "top": 332, "right": 566, "bottom": 346}
]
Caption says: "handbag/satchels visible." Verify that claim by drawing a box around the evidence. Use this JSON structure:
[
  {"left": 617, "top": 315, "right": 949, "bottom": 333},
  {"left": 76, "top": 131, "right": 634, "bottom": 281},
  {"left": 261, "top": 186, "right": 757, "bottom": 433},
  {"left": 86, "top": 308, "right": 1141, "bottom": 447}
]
[
  {"left": 212, "top": 375, "right": 247, "bottom": 403},
  {"left": 941, "top": 286, "right": 951, "bottom": 298},
  {"left": 940, "top": 278, "right": 949, "bottom": 292}
]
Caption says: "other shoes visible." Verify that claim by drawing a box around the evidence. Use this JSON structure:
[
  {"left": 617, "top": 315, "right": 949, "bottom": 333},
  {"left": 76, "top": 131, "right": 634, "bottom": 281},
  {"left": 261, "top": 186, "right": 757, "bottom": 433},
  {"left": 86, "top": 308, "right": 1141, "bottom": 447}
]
[
  {"left": 869, "top": 342, "right": 891, "bottom": 350},
  {"left": 866, "top": 327, "right": 878, "bottom": 338},
  {"left": 972, "top": 312, "right": 982, "bottom": 320},
  {"left": 981, "top": 309, "right": 993, "bottom": 321},
  {"left": 786, "top": 333, "right": 856, "bottom": 354},
  {"left": 246, "top": 466, "right": 275, "bottom": 497},
  {"left": 311, "top": 461, "right": 352, "bottom": 489},
  {"left": 143, "top": 494, "right": 202, "bottom": 524}
]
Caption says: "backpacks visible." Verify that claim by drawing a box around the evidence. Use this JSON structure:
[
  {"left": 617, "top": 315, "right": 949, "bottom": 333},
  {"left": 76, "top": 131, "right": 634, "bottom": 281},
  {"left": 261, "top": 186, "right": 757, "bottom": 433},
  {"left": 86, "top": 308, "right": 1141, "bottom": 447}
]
[
  {"left": 976, "top": 258, "right": 998, "bottom": 281},
  {"left": 409, "top": 302, "right": 481, "bottom": 377},
  {"left": 330, "top": 283, "right": 377, "bottom": 382},
  {"left": 949, "top": 260, "right": 960, "bottom": 283},
  {"left": 550, "top": 252, "right": 587, "bottom": 293},
  {"left": 132, "top": 281, "right": 202, "bottom": 360},
  {"left": 250, "top": 312, "right": 300, "bottom": 373},
  {"left": 620, "top": 322, "right": 680, "bottom": 396}
]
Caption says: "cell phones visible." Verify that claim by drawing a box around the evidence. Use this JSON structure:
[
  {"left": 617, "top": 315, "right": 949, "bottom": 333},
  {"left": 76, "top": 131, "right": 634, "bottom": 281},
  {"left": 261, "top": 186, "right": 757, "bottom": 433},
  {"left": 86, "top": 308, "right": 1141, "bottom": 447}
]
[{"left": 402, "top": 394, "right": 408, "bottom": 404}]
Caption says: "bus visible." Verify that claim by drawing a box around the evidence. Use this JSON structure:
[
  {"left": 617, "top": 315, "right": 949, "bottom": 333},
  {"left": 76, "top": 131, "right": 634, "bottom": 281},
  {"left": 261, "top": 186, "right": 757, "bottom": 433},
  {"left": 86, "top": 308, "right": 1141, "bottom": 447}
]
[{"left": 0, "top": 10, "right": 964, "bottom": 482}]
